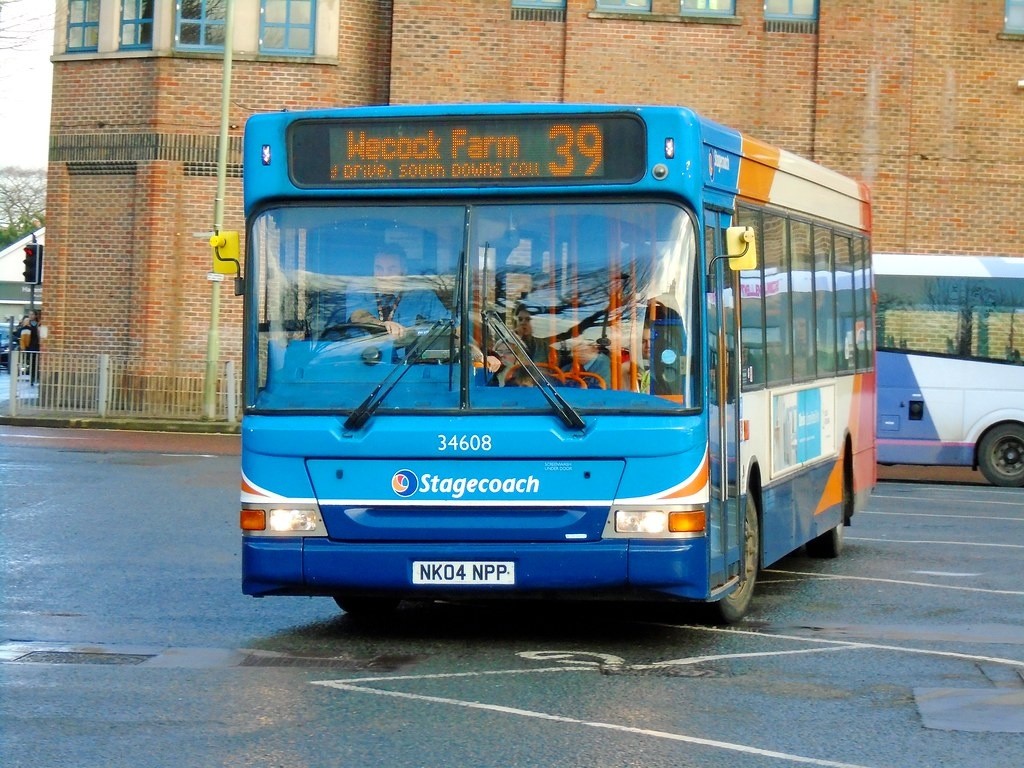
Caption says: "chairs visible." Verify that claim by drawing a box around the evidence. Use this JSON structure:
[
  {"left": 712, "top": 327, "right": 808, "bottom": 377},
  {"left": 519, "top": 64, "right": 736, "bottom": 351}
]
[
  {"left": 885, "top": 336, "right": 909, "bottom": 349},
  {"left": 947, "top": 338, "right": 961, "bottom": 354},
  {"left": 1005, "top": 346, "right": 1021, "bottom": 360}
]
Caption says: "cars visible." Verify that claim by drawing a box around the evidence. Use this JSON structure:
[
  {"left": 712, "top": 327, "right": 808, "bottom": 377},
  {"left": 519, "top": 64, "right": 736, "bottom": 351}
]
[{"left": 0, "top": 323, "right": 18, "bottom": 367}]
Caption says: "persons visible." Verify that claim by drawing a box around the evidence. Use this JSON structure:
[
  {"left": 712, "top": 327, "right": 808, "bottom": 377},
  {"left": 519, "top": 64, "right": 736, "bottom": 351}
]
[
  {"left": 12, "top": 309, "right": 39, "bottom": 385},
  {"left": 512, "top": 304, "right": 550, "bottom": 366},
  {"left": 346, "top": 243, "right": 503, "bottom": 373},
  {"left": 574, "top": 323, "right": 664, "bottom": 390}
]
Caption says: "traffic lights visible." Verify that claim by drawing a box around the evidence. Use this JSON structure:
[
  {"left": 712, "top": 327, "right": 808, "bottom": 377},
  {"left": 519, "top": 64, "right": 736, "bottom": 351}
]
[{"left": 23, "top": 242, "right": 43, "bottom": 285}]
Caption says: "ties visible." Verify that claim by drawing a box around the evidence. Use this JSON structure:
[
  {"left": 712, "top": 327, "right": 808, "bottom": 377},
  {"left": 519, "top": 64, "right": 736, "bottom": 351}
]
[{"left": 382, "top": 295, "right": 393, "bottom": 321}]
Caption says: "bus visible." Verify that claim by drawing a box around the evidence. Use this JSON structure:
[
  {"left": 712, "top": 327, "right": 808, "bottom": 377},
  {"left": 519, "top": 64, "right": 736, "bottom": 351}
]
[
  {"left": 869, "top": 251, "right": 1024, "bottom": 487},
  {"left": 211, "top": 102, "right": 880, "bottom": 635}
]
